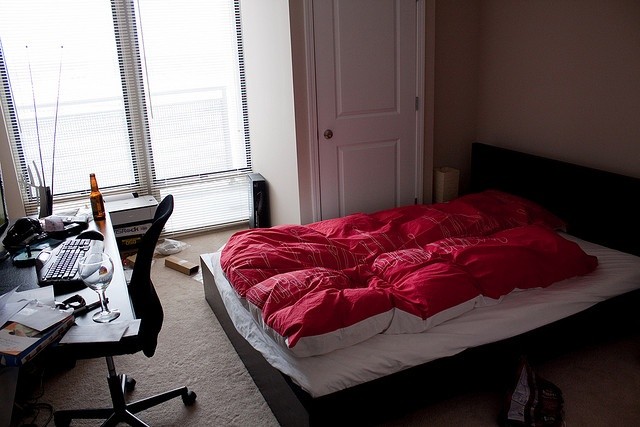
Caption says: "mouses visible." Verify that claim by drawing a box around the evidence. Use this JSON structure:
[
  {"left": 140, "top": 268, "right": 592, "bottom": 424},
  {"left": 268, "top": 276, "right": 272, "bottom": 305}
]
[{"left": 77, "top": 229, "right": 105, "bottom": 240}]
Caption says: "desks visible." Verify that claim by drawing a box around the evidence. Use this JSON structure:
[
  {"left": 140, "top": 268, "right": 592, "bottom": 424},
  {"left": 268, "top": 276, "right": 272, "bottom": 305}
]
[{"left": 0, "top": 203, "right": 140, "bottom": 371}]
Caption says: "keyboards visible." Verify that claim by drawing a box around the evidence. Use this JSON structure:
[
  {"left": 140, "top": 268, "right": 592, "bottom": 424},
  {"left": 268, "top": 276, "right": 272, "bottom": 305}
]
[{"left": 39, "top": 238, "right": 105, "bottom": 284}]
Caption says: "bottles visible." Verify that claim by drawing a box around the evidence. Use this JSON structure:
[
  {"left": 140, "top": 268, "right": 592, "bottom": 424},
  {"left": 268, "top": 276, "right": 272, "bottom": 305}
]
[{"left": 89, "top": 173, "right": 106, "bottom": 221}]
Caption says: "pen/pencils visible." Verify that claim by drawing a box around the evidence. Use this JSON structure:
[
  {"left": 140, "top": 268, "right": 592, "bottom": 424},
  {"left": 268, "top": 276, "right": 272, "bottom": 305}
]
[{"left": 72, "top": 298, "right": 108, "bottom": 314}]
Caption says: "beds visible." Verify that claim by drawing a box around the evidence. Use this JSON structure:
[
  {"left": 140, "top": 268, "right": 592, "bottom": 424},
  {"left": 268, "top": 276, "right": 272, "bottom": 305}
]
[{"left": 196, "top": 141, "right": 639, "bottom": 426}]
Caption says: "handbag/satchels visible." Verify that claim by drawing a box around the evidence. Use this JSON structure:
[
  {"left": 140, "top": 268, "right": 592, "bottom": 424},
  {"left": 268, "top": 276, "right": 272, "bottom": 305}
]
[{"left": 501, "top": 357, "right": 566, "bottom": 427}]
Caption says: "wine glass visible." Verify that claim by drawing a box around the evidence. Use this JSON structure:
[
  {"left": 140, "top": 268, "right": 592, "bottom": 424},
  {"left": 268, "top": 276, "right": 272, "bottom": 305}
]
[{"left": 78, "top": 252, "right": 120, "bottom": 323}]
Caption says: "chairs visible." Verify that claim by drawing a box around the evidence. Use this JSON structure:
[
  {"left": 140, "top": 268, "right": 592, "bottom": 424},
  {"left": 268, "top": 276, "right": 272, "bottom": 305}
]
[{"left": 49, "top": 191, "right": 198, "bottom": 427}]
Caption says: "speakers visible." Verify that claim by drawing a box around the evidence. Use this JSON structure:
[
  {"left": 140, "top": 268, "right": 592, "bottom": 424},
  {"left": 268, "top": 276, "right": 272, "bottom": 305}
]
[{"left": 247, "top": 173, "right": 271, "bottom": 227}]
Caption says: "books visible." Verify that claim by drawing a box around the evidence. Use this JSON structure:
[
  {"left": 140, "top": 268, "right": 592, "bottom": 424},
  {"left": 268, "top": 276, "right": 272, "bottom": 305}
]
[{"left": 0, "top": 312, "right": 75, "bottom": 367}]
